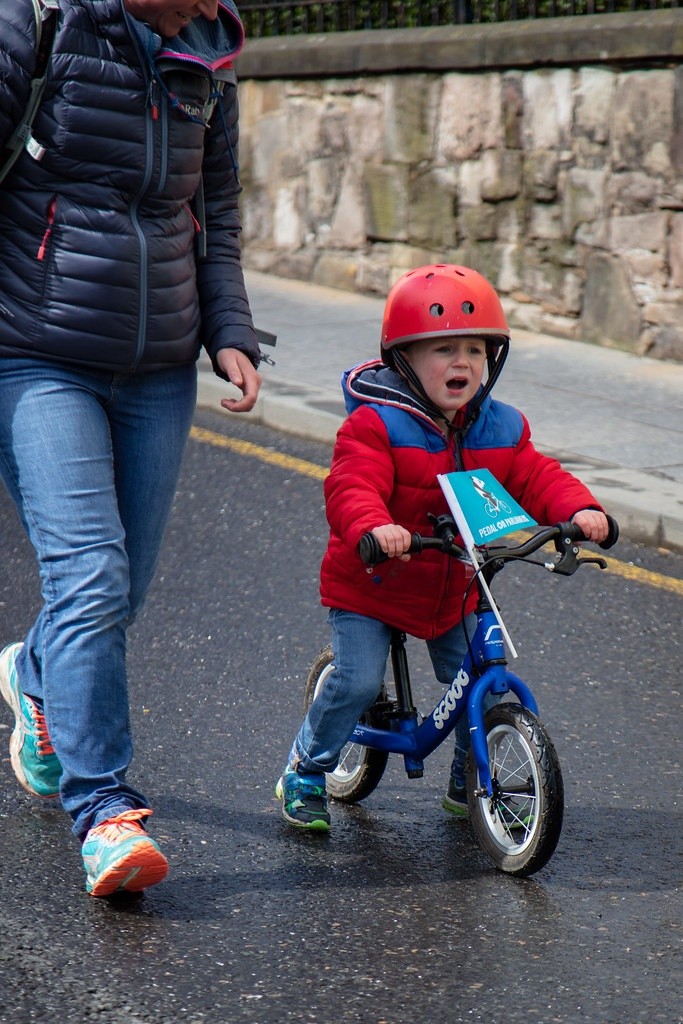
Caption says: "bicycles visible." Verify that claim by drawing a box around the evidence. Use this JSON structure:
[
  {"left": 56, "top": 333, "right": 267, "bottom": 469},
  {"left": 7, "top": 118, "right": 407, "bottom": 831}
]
[{"left": 300, "top": 514, "right": 619, "bottom": 870}]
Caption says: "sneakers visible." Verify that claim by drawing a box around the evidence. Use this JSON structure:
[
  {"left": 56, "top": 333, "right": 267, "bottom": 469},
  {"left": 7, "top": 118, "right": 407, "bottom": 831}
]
[
  {"left": 275, "top": 762, "right": 331, "bottom": 830},
  {"left": 442, "top": 776, "right": 534, "bottom": 828},
  {"left": 0, "top": 641, "right": 63, "bottom": 798},
  {"left": 81, "top": 809, "right": 168, "bottom": 896}
]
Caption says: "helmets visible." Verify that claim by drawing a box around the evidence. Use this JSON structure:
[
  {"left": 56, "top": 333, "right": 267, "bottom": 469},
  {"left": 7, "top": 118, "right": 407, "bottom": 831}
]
[{"left": 381, "top": 264, "right": 510, "bottom": 364}]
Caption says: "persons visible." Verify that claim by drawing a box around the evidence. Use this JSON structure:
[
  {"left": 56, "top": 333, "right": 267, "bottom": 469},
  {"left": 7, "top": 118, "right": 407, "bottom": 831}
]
[
  {"left": 0, "top": 0, "right": 261, "bottom": 894},
  {"left": 276, "top": 265, "right": 609, "bottom": 831}
]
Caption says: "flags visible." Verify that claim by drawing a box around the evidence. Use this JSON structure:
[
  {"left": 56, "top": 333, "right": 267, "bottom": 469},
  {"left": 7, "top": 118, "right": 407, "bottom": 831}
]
[{"left": 441, "top": 468, "right": 538, "bottom": 546}]
